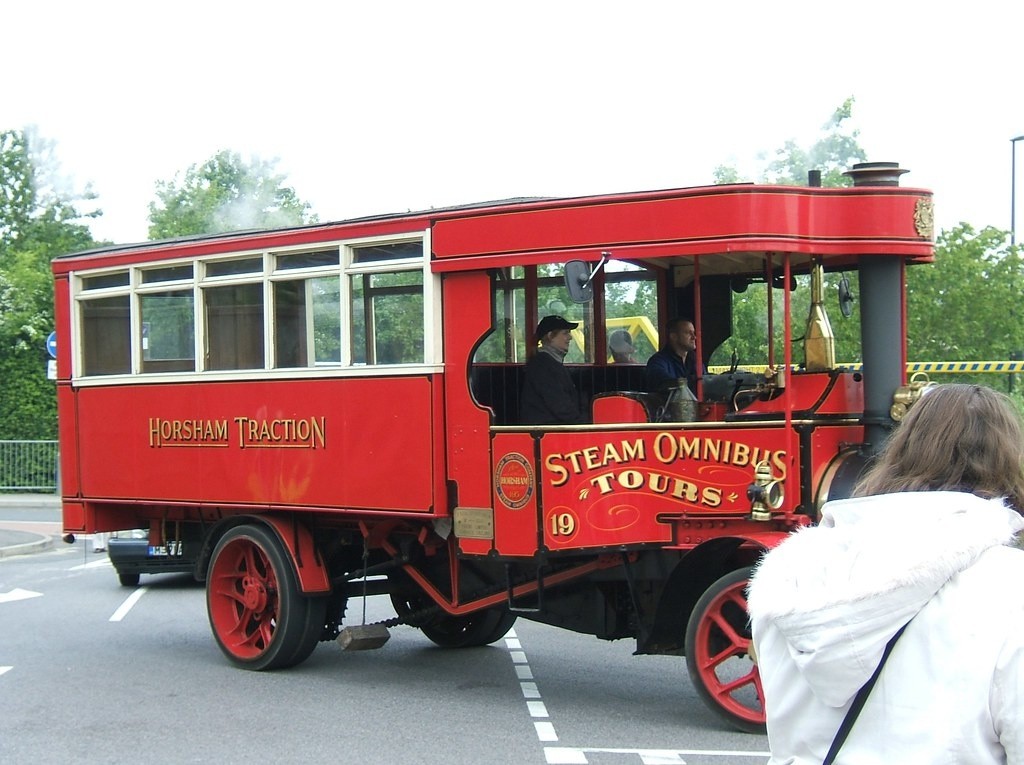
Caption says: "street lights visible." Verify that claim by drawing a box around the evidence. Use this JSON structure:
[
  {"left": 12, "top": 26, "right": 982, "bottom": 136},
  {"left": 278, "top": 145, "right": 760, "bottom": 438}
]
[{"left": 1010, "top": 135, "right": 1024, "bottom": 395}]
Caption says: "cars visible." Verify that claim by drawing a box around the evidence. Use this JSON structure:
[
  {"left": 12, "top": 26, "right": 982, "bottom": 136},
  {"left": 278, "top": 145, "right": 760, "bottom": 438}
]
[{"left": 107, "top": 529, "right": 205, "bottom": 586}]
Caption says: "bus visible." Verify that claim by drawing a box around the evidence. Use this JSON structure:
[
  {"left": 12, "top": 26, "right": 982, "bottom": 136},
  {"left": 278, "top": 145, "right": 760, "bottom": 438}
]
[{"left": 49, "top": 161, "right": 937, "bottom": 736}]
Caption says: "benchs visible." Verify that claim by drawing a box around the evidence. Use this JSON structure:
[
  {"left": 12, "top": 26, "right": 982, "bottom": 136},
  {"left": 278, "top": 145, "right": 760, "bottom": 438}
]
[{"left": 589, "top": 391, "right": 669, "bottom": 424}]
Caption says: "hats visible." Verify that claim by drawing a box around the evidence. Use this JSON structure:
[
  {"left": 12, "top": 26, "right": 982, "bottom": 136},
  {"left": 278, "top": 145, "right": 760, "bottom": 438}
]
[{"left": 535, "top": 315, "right": 579, "bottom": 340}]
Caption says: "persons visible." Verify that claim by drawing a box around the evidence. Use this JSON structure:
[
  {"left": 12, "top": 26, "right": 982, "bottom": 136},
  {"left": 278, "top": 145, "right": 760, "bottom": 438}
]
[
  {"left": 644, "top": 317, "right": 712, "bottom": 415},
  {"left": 609, "top": 329, "right": 639, "bottom": 364},
  {"left": 519, "top": 315, "right": 582, "bottom": 424},
  {"left": 746, "top": 383, "right": 1024, "bottom": 765}
]
